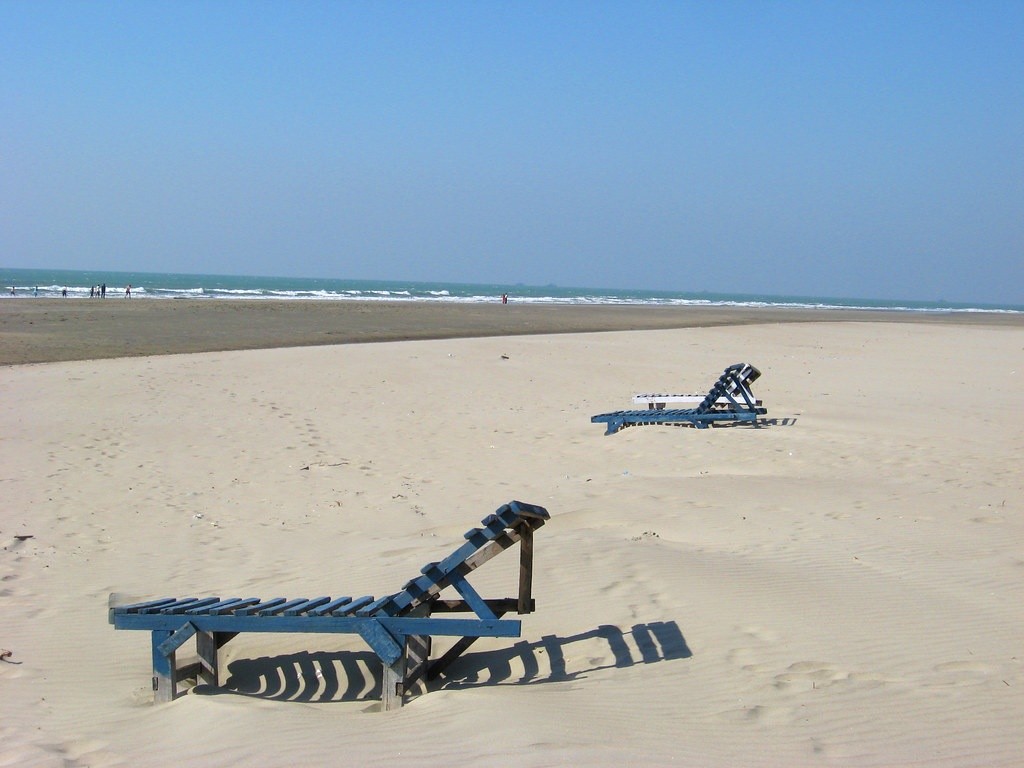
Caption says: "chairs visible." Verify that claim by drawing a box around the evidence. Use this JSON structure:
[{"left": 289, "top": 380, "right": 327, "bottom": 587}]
[
  {"left": 590, "top": 363, "right": 767, "bottom": 434},
  {"left": 108, "top": 499, "right": 554, "bottom": 713}
]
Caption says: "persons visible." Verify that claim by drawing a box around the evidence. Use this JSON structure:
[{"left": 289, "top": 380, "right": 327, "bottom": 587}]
[
  {"left": 62, "top": 285, "right": 67, "bottom": 298},
  {"left": 124, "top": 285, "right": 131, "bottom": 299},
  {"left": 90, "top": 284, "right": 105, "bottom": 299},
  {"left": 503, "top": 293, "right": 507, "bottom": 304},
  {"left": 11, "top": 286, "right": 15, "bottom": 297},
  {"left": 34, "top": 286, "right": 38, "bottom": 297}
]
